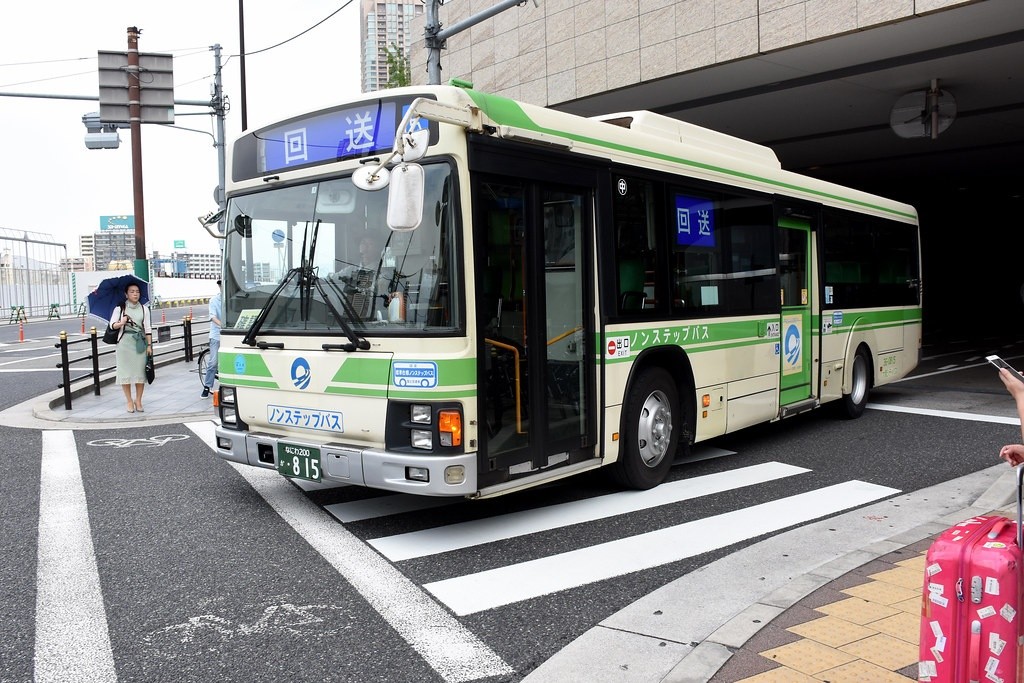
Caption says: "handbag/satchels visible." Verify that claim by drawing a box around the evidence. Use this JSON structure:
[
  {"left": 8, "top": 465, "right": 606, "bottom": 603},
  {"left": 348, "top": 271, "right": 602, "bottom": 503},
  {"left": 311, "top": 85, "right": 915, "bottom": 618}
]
[
  {"left": 146, "top": 353, "right": 155, "bottom": 384},
  {"left": 102, "top": 324, "right": 120, "bottom": 344}
]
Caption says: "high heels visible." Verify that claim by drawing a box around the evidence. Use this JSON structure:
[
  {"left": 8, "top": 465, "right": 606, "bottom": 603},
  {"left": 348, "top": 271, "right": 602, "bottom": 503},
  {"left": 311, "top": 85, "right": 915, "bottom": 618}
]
[
  {"left": 127, "top": 401, "right": 134, "bottom": 413},
  {"left": 134, "top": 400, "right": 144, "bottom": 411}
]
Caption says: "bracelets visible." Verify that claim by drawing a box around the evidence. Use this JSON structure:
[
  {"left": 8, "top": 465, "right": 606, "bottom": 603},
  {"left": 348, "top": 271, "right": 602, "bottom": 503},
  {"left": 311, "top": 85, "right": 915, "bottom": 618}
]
[{"left": 148, "top": 344, "right": 151, "bottom": 346}]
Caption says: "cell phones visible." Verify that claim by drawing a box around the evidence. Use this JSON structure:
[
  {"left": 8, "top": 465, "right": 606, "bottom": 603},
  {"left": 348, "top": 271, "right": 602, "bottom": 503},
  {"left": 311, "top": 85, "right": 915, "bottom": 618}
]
[{"left": 986, "top": 355, "right": 1024, "bottom": 383}]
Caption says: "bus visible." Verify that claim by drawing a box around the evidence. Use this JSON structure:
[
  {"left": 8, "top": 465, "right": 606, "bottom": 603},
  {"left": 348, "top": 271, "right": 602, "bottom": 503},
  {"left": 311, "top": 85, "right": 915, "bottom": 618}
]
[{"left": 197, "top": 79, "right": 923, "bottom": 500}]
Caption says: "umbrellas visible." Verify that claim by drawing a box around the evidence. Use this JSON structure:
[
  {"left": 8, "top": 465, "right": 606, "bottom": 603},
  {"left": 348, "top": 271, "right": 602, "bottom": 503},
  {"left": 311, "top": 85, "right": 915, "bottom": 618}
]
[{"left": 83, "top": 273, "right": 152, "bottom": 324}]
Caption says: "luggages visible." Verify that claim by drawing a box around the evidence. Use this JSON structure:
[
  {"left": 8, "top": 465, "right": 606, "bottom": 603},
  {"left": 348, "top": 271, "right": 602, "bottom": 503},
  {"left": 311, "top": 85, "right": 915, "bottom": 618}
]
[{"left": 918, "top": 464, "right": 1024, "bottom": 683}]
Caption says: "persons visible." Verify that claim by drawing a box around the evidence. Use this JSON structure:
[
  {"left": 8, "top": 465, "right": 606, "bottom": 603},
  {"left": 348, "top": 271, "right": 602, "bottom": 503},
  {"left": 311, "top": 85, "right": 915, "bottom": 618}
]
[
  {"left": 200, "top": 280, "right": 222, "bottom": 400},
  {"left": 998, "top": 367, "right": 1024, "bottom": 467},
  {"left": 318, "top": 228, "right": 388, "bottom": 297},
  {"left": 109, "top": 282, "right": 152, "bottom": 413}
]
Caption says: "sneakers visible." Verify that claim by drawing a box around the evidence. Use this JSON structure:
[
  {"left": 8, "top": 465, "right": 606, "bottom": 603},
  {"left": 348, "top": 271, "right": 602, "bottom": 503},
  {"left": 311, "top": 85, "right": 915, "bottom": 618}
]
[{"left": 200, "top": 386, "right": 210, "bottom": 398}]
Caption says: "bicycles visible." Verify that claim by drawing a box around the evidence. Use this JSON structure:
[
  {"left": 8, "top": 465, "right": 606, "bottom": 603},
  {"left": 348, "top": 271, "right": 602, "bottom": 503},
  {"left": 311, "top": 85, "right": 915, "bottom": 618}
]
[{"left": 189, "top": 344, "right": 218, "bottom": 394}]
